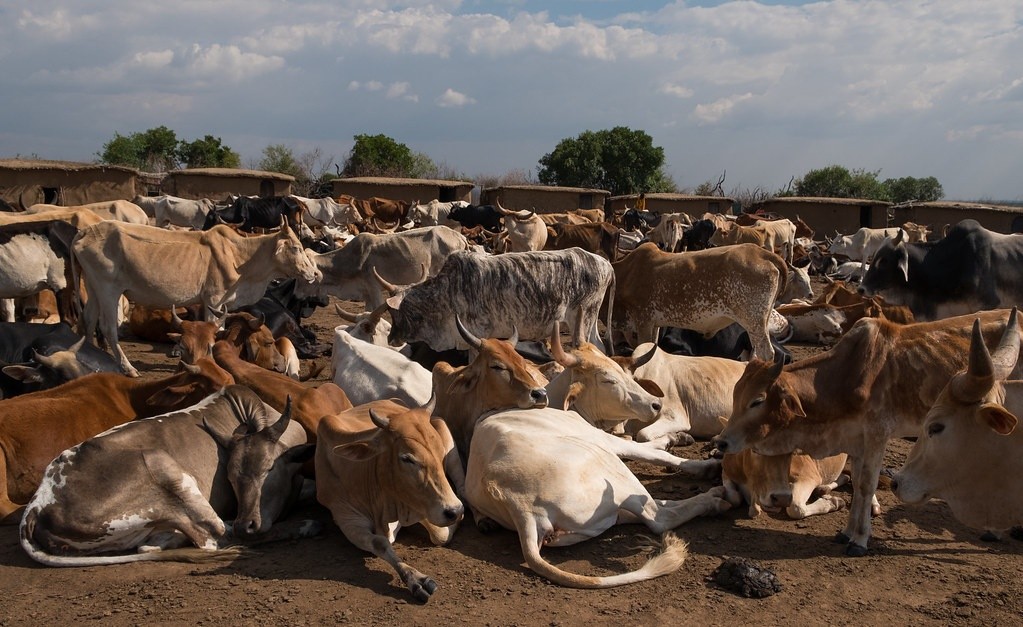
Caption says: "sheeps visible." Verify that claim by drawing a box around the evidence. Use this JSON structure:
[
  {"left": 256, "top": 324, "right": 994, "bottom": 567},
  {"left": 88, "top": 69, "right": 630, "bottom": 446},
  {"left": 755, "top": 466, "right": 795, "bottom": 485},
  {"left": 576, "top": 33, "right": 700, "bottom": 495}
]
[{"left": 428, "top": 312, "right": 563, "bottom": 442}]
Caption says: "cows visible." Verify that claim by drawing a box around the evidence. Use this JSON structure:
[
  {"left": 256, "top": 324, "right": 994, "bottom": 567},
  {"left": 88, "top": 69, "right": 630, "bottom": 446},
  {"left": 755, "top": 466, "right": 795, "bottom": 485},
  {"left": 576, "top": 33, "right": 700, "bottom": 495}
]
[
  {"left": 0, "top": 185, "right": 1023, "bottom": 382},
  {"left": 316, "top": 396, "right": 466, "bottom": 605},
  {"left": 463, "top": 407, "right": 729, "bottom": 589},
  {"left": 548, "top": 303, "right": 764, "bottom": 448},
  {"left": 886, "top": 304, "right": 1023, "bottom": 535},
  {"left": 0, "top": 306, "right": 438, "bottom": 568},
  {"left": 715, "top": 309, "right": 1023, "bottom": 555}
]
